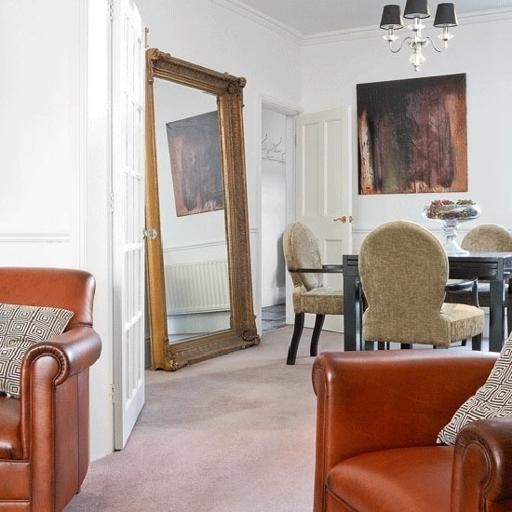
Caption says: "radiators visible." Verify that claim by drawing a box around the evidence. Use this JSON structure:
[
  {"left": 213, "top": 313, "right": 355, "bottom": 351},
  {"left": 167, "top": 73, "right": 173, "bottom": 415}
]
[{"left": 162, "top": 259, "right": 231, "bottom": 317}]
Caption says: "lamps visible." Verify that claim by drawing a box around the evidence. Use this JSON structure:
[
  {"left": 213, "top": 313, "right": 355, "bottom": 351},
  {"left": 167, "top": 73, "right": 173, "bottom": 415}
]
[{"left": 380, "top": 0, "right": 457, "bottom": 73}]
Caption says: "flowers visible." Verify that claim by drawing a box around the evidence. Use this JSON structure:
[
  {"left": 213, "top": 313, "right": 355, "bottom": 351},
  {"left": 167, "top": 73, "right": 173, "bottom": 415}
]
[{"left": 424, "top": 200, "right": 479, "bottom": 238}]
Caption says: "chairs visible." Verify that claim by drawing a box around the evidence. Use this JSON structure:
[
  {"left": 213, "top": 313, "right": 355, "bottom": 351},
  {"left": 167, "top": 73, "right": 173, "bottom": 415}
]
[
  {"left": 356, "top": 220, "right": 483, "bottom": 350},
  {"left": 0, "top": 266, "right": 102, "bottom": 512},
  {"left": 283, "top": 221, "right": 344, "bottom": 365},
  {"left": 312, "top": 334, "right": 512, "bottom": 512},
  {"left": 433, "top": 224, "right": 512, "bottom": 350}
]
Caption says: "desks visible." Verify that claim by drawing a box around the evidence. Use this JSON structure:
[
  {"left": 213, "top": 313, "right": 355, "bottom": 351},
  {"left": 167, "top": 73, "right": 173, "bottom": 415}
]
[{"left": 342, "top": 252, "right": 511, "bottom": 352}]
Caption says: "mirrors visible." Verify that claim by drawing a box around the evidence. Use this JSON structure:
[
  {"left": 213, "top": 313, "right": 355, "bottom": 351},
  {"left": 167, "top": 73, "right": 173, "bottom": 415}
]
[{"left": 145, "top": 49, "right": 261, "bottom": 372}]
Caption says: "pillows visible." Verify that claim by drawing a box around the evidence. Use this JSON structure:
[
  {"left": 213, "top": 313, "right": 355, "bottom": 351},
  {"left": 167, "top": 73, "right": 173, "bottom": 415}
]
[
  {"left": 0, "top": 304, "right": 74, "bottom": 399},
  {"left": 437, "top": 335, "right": 512, "bottom": 446}
]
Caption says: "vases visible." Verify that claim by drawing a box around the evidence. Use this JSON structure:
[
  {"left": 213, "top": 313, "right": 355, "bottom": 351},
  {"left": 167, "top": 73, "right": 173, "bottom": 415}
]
[{"left": 442, "top": 226, "right": 470, "bottom": 257}]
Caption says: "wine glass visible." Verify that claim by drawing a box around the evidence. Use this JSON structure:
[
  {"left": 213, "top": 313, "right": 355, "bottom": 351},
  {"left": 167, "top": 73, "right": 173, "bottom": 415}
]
[{"left": 423, "top": 204, "right": 480, "bottom": 255}]
